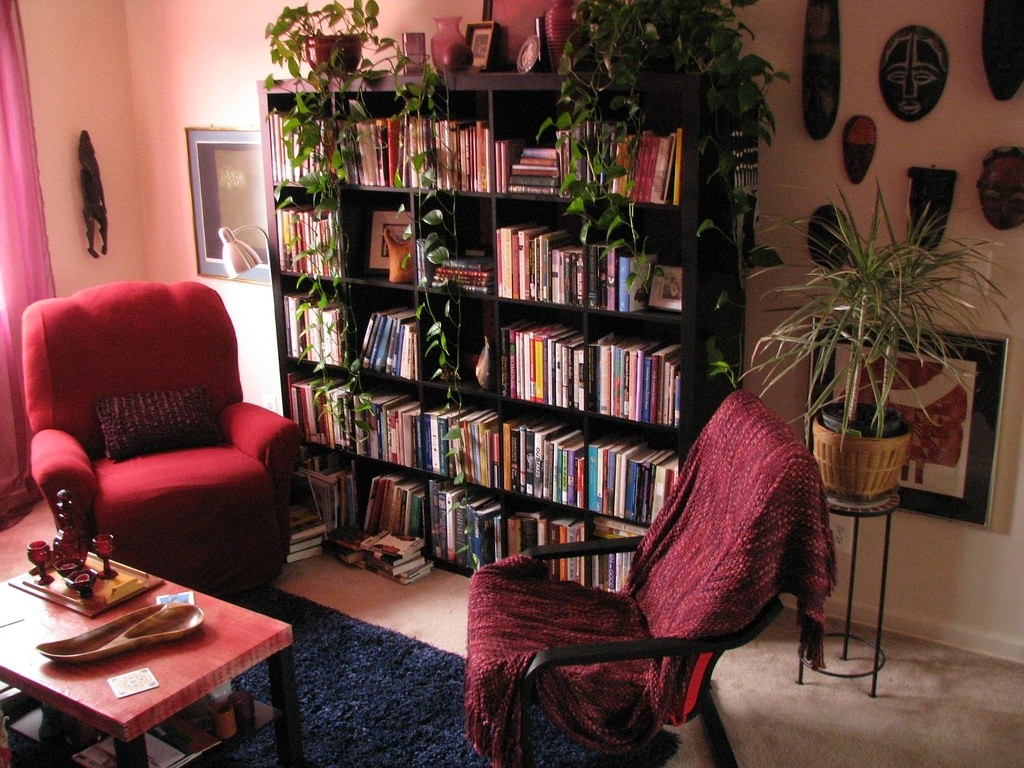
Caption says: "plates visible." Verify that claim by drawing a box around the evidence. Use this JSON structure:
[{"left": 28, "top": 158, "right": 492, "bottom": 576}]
[{"left": 516, "top": 35, "right": 540, "bottom": 74}]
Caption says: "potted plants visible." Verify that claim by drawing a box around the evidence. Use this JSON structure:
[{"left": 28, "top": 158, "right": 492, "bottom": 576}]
[
  {"left": 739, "top": 173, "right": 1017, "bottom": 506},
  {"left": 262, "top": 0, "right": 490, "bottom": 584},
  {"left": 534, "top": 0, "right": 793, "bottom": 309}
]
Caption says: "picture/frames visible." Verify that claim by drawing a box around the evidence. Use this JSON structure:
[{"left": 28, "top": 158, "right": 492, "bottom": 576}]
[
  {"left": 182, "top": 125, "right": 274, "bottom": 286},
  {"left": 463, "top": 22, "right": 501, "bottom": 74},
  {"left": 806, "top": 313, "right": 1011, "bottom": 530}
]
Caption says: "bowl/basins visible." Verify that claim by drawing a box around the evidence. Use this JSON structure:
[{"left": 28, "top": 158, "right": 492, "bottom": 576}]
[{"left": 54, "top": 557, "right": 85, "bottom": 578}]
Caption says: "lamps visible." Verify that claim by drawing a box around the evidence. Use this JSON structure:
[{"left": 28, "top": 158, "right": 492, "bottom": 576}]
[{"left": 217, "top": 224, "right": 269, "bottom": 282}]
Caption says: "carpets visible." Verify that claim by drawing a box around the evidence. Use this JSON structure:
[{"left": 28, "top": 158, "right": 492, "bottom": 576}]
[{"left": 4, "top": 584, "right": 683, "bottom": 768}]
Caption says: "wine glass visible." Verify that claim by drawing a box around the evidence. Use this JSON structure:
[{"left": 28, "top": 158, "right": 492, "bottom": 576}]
[
  {"left": 27, "top": 540, "right": 54, "bottom": 586},
  {"left": 92, "top": 534, "right": 118, "bottom": 579}
]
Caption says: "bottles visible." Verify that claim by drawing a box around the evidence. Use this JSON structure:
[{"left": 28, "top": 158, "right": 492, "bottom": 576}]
[
  {"left": 430, "top": 16, "right": 469, "bottom": 75},
  {"left": 546, "top": 0, "right": 578, "bottom": 74},
  {"left": 478, "top": 333, "right": 501, "bottom": 392},
  {"left": 54, "top": 489, "right": 88, "bottom": 564}
]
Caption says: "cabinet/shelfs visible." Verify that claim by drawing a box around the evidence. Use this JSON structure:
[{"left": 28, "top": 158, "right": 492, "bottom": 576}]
[
  {"left": 795, "top": 493, "right": 901, "bottom": 696},
  {"left": 256, "top": 66, "right": 731, "bottom": 589}
]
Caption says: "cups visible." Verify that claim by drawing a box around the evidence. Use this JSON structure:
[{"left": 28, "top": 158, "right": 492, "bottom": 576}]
[
  {"left": 209, "top": 702, "right": 236, "bottom": 739},
  {"left": 64, "top": 568, "right": 98, "bottom": 599},
  {"left": 228, "top": 691, "right": 255, "bottom": 725},
  {"left": 401, "top": 32, "right": 426, "bottom": 76}
]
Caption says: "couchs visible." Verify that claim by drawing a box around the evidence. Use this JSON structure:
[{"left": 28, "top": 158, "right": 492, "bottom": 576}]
[{"left": 23, "top": 279, "right": 304, "bottom": 608}]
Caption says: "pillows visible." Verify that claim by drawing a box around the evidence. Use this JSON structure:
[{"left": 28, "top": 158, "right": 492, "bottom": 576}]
[{"left": 94, "top": 384, "right": 225, "bottom": 460}]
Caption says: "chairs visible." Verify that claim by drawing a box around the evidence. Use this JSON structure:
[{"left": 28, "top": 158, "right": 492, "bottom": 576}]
[{"left": 460, "top": 391, "right": 839, "bottom": 768}]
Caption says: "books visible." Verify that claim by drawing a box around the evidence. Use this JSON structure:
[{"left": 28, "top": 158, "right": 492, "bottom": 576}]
[{"left": 268, "top": 105, "right": 682, "bottom": 586}]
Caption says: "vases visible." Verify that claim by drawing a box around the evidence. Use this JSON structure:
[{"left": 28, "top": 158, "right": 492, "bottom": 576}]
[
  {"left": 431, "top": 16, "right": 467, "bottom": 75},
  {"left": 547, "top": 1, "right": 580, "bottom": 73}
]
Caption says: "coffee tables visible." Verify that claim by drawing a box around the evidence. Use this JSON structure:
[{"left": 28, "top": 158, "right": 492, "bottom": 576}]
[{"left": 0, "top": 553, "right": 301, "bottom": 768}]
